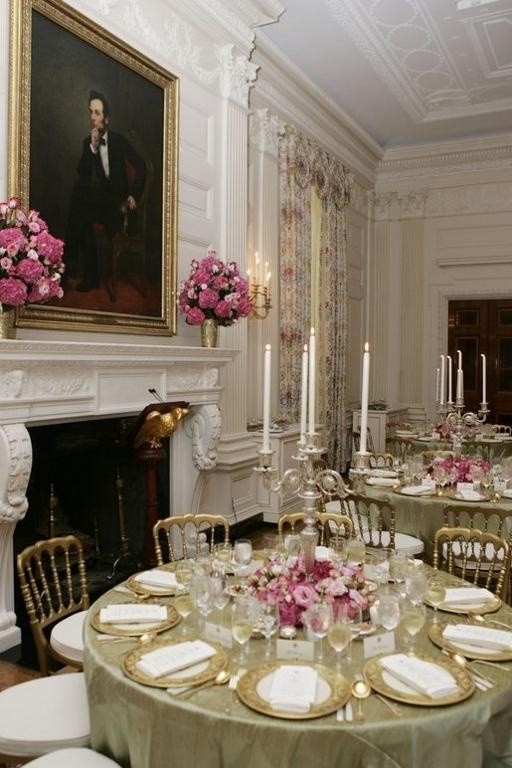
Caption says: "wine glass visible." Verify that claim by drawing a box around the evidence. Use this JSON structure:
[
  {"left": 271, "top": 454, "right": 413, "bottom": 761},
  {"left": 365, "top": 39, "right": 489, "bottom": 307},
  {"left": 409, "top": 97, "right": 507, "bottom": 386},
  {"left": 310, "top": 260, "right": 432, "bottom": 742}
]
[
  {"left": 393, "top": 417, "right": 512, "bottom": 445},
  {"left": 365, "top": 447, "right": 511, "bottom": 505},
  {"left": 188, "top": 530, "right": 447, "bottom": 666}
]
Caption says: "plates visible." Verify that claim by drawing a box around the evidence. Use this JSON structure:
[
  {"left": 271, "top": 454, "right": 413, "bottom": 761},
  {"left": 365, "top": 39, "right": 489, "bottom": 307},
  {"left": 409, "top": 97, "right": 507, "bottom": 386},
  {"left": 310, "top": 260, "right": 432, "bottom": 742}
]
[
  {"left": 86, "top": 564, "right": 231, "bottom": 704},
  {"left": 428, "top": 579, "right": 512, "bottom": 670},
  {"left": 234, "top": 659, "right": 354, "bottom": 723},
  {"left": 362, "top": 652, "right": 477, "bottom": 709}
]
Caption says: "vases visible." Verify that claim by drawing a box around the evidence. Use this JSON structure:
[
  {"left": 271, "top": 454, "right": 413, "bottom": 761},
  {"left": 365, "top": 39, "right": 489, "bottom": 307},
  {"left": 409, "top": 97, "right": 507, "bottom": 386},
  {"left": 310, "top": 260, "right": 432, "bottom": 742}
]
[
  {"left": 2, "top": 303, "right": 17, "bottom": 340},
  {"left": 199, "top": 314, "right": 219, "bottom": 348}
]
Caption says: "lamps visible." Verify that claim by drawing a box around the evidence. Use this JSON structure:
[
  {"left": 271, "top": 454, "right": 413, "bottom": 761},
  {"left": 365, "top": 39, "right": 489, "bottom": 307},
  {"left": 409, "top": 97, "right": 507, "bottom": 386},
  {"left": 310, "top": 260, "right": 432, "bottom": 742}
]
[{"left": 242, "top": 247, "right": 274, "bottom": 320}]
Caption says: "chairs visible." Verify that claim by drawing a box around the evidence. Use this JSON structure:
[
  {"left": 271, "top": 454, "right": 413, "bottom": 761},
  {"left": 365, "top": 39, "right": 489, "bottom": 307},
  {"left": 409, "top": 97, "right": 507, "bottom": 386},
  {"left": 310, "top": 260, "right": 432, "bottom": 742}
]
[
  {"left": 324, "top": 419, "right": 512, "bottom": 602},
  {"left": 0, "top": 510, "right": 512, "bottom": 768}
]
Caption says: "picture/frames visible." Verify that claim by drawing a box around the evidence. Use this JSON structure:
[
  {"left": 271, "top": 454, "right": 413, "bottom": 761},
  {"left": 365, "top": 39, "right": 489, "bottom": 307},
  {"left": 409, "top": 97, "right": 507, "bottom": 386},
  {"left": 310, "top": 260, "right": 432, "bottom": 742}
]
[{"left": 5, "top": 0, "right": 182, "bottom": 343}]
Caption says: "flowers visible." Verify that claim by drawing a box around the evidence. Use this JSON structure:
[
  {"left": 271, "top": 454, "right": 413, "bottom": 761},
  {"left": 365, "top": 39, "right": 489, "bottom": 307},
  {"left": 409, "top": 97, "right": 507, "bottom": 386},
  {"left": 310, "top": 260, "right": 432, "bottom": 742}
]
[
  {"left": 178, "top": 243, "right": 253, "bottom": 323},
  {"left": 0, "top": 192, "right": 64, "bottom": 309}
]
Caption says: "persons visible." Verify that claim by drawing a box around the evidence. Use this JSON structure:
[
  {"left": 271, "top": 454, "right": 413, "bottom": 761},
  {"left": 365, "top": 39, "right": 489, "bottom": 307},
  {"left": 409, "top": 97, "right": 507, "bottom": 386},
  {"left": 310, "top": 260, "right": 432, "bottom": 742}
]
[{"left": 64, "top": 90, "right": 147, "bottom": 292}]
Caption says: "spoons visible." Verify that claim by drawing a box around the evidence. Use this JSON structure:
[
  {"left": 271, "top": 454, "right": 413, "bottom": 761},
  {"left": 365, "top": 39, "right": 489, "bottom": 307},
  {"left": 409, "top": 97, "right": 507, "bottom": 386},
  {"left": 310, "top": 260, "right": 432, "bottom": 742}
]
[{"left": 351, "top": 680, "right": 372, "bottom": 724}]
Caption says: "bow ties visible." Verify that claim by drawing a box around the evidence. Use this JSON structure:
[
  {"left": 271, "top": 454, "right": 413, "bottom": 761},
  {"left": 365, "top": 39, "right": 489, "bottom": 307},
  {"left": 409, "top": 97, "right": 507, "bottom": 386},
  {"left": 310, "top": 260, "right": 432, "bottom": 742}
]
[{"left": 99, "top": 138, "right": 105, "bottom": 145}]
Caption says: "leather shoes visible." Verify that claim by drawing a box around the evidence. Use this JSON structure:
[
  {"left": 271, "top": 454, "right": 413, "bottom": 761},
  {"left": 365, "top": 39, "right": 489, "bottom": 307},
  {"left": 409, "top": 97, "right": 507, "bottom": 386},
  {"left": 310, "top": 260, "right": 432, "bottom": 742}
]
[{"left": 75, "top": 275, "right": 101, "bottom": 292}]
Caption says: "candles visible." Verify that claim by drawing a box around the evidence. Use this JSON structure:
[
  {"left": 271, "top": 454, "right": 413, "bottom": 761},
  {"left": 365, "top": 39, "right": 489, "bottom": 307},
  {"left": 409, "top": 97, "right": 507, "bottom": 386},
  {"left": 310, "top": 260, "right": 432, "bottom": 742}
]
[
  {"left": 258, "top": 326, "right": 373, "bottom": 463},
  {"left": 435, "top": 347, "right": 490, "bottom": 412}
]
